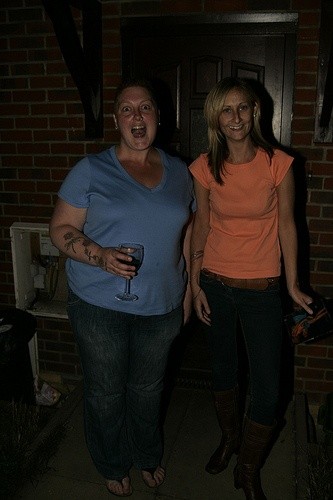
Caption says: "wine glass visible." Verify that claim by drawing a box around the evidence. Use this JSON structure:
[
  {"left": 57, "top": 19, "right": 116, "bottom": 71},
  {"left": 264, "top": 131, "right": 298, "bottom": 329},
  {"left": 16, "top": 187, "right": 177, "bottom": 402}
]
[{"left": 115, "top": 243, "right": 144, "bottom": 302}]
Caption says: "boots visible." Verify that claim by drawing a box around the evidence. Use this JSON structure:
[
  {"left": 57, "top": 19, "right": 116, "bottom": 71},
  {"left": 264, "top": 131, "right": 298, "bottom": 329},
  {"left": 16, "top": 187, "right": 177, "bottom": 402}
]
[
  {"left": 234, "top": 414, "right": 284, "bottom": 500},
  {"left": 206, "top": 380, "right": 248, "bottom": 474}
]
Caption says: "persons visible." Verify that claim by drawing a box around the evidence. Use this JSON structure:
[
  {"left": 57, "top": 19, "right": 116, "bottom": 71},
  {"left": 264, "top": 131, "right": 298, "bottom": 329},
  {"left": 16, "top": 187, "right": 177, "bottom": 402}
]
[
  {"left": 186, "top": 76, "right": 314, "bottom": 500},
  {"left": 48, "top": 79, "right": 198, "bottom": 496}
]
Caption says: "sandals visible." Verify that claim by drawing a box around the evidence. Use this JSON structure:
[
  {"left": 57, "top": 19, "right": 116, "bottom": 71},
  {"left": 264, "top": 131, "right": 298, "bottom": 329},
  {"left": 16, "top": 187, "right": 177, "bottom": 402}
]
[
  {"left": 140, "top": 465, "right": 165, "bottom": 487},
  {"left": 106, "top": 476, "right": 133, "bottom": 496}
]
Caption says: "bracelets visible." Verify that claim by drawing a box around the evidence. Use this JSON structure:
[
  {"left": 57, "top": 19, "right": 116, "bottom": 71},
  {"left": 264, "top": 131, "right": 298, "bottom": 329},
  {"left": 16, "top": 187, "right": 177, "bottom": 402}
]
[
  {"left": 192, "top": 288, "right": 202, "bottom": 302},
  {"left": 189, "top": 250, "right": 204, "bottom": 268}
]
